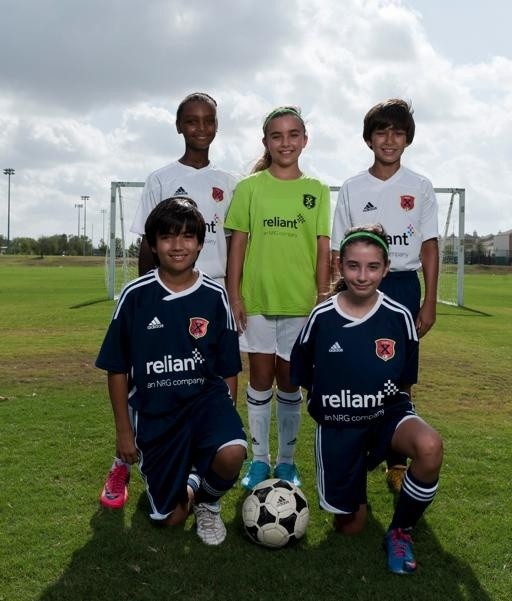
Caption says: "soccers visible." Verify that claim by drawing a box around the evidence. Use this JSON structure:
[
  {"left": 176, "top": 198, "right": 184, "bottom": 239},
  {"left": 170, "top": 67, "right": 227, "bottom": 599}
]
[{"left": 240, "top": 478, "right": 310, "bottom": 550}]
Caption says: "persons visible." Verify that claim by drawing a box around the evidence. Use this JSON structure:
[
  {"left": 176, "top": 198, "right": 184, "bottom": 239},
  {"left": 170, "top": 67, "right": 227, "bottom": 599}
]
[
  {"left": 98, "top": 92, "right": 246, "bottom": 508},
  {"left": 288, "top": 229, "right": 444, "bottom": 576},
  {"left": 225, "top": 105, "right": 335, "bottom": 493},
  {"left": 93, "top": 198, "right": 247, "bottom": 546},
  {"left": 331, "top": 98, "right": 441, "bottom": 494}
]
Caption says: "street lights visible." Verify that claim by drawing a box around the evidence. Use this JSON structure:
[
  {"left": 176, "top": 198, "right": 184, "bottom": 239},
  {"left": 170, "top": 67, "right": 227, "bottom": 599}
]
[
  {"left": 69, "top": 193, "right": 110, "bottom": 244},
  {"left": 3, "top": 161, "right": 16, "bottom": 245}
]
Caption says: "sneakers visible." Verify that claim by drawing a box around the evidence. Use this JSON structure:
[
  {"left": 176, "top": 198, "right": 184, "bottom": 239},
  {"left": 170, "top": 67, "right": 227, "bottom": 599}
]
[
  {"left": 385, "top": 465, "right": 409, "bottom": 494},
  {"left": 193, "top": 502, "right": 227, "bottom": 546},
  {"left": 384, "top": 526, "right": 417, "bottom": 575},
  {"left": 240, "top": 460, "right": 303, "bottom": 492},
  {"left": 99, "top": 456, "right": 131, "bottom": 509}
]
